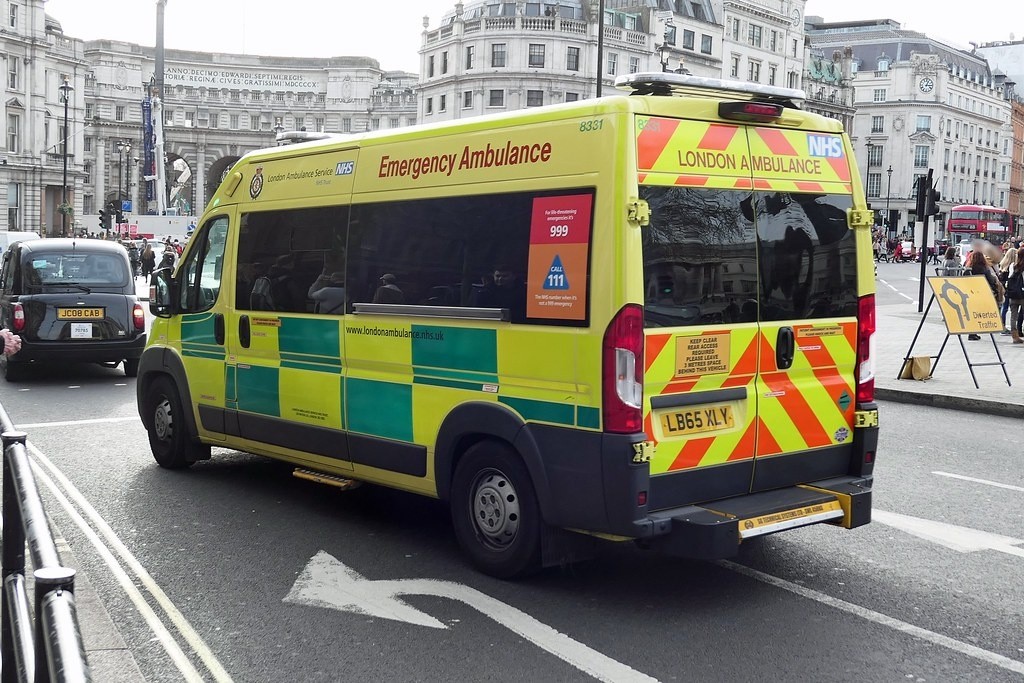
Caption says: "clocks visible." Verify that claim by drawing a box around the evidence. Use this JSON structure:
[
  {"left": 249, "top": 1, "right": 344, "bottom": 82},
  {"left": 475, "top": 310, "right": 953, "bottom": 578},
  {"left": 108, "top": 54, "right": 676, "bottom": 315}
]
[{"left": 919, "top": 78, "right": 934, "bottom": 93}]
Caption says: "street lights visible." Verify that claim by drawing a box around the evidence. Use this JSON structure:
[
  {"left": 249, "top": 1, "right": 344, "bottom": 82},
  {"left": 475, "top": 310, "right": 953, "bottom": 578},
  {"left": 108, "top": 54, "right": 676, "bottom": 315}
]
[
  {"left": 117, "top": 140, "right": 125, "bottom": 199},
  {"left": 885, "top": 166, "right": 893, "bottom": 236},
  {"left": 59, "top": 79, "right": 73, "bottom": 237},
  {"left": 125, "top": 142, "right": 131, "bottom": 199}
]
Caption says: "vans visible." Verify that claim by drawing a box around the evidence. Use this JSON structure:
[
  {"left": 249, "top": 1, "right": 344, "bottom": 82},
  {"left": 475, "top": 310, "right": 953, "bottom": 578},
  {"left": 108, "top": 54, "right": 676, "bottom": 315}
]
[{"left": 136, "top": 71, "right": 879, "bottom": 577}]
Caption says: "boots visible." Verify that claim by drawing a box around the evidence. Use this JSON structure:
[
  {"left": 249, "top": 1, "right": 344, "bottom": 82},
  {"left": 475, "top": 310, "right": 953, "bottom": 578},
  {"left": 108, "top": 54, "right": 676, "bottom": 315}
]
[{"left": 1011, "top": 329, "right": 1024, "bottom": 344}]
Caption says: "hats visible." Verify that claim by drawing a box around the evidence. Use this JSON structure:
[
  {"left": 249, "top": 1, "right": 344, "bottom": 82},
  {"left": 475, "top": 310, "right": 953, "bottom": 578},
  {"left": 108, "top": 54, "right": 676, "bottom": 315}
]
[{"left": 379, "top": 273, "right": 396, "bottom": 280}]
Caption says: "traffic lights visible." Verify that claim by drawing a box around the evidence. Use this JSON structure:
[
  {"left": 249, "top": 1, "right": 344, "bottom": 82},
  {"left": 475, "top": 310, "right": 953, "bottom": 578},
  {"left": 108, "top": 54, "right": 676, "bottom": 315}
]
[
  {"left": 913, "top": 175, "right": 941, "bottom": 222},
  {"left": 99, "top": 208, "right": 111, "bottom": 229}
]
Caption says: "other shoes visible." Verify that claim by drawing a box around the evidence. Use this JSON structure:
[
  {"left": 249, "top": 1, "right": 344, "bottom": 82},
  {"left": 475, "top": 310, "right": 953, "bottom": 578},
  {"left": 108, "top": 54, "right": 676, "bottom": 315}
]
[
  {"left": 1000, "top": 329, "right": 1012, "bottom": 336},
  {"left": 968, "top": 334, "right": 981, "bottom": 340},
  {"left": 1018, "top": 330, "right": 1024, "bottom": 336}
]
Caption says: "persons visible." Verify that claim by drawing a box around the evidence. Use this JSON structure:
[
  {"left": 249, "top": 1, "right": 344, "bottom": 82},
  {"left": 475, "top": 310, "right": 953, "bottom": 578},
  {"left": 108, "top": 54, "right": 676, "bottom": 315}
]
[
  {"left": 251, "top": 253, "right": 528, "bottom": 324},
  {"left": 56, "top": 220, "right": 195, "bottom": 283},
  {"left": 722, "top": 298, "right": 740, "bottom": 323},
  {"left": 0, "top": 327, "right": 22, "bottom": 357},
  {"left": 872, "top": 226, "right": 1024, "bottom": 344}
]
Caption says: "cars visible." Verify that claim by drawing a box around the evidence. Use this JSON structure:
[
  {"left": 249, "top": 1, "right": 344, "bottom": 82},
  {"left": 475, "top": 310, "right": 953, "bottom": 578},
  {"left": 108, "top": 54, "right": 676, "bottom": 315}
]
[
  {"left": 126, "top": 222, "right": 227, "bottom": 276},
  {"left": 889, "top": 238, "right": 977, "bottom": 268},
  {"left": 1, "top": 237, "right": 146, "bottom": 383}
]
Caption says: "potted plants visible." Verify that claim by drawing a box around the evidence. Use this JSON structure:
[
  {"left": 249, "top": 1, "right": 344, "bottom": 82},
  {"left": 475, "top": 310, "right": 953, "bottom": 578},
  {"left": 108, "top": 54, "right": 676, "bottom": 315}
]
[{"left": 57, "top": 203, "right": 72, "bottom": 215}]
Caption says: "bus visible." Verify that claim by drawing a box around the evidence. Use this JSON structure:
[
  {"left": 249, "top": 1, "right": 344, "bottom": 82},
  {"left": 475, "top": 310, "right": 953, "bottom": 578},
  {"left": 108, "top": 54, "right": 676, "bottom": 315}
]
[{"left": 948, "top": 205, "right": 1013, "bottom": 257}]
